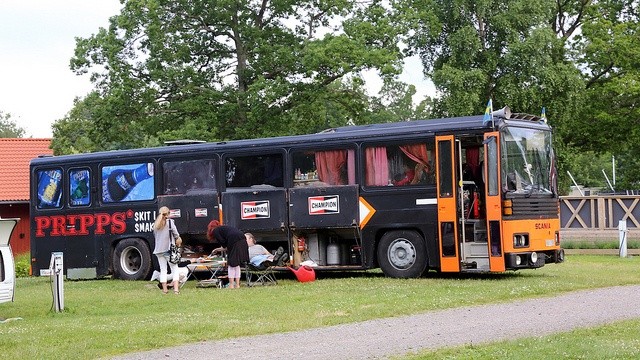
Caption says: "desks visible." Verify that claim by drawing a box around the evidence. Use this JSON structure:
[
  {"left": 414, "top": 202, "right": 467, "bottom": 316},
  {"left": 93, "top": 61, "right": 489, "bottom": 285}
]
[{"left": 179, "top": 254, "right": 227, "bottom": 289}]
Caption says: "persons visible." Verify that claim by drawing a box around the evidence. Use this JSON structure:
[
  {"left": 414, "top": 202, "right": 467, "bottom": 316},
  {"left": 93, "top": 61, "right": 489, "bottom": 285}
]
[
  {"left": 390, "top": 156, "right": 415, "bottom": 187},
  {"left": 152, "top": 207, "right": 182, "bottom": 296},
  {"left": 207, "top": 220, "right": 250, "bottom": 289},
  {"left": 212, "top": 233, "right": 288, "bottom": 271}
]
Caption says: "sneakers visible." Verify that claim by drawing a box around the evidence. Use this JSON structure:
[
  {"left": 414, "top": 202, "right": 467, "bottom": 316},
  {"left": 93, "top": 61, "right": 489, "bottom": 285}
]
[
  {"left": 278, "top": 253, "right": 288, "bottom": 266},
  {"left": 274, "top": 246, "right": 284, "bottom": 261}
]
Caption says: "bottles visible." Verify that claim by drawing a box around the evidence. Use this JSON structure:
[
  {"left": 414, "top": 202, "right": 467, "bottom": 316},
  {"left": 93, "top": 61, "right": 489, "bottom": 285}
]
[
  {"left": 102, "top": 163, "right": 154, "bottom": 205},
  {"left": 37, "top": 169, "right": 63, "bottom": 209}
]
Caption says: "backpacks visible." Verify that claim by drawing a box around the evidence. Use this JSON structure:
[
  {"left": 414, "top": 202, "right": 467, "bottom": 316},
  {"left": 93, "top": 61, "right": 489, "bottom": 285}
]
[{"left": 170, "top": 245, "right": 182, "bottom": 264}]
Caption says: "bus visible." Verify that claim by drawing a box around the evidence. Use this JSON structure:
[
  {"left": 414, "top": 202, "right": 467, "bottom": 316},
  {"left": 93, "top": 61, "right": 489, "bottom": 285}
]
[{"left": 28, "top": 96, "right": 566, "bottom": 280}]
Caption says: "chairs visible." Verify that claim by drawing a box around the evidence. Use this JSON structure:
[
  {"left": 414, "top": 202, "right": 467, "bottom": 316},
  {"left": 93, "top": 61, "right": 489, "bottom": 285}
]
[{"left": 245, "top": 260, "right": 278, "bottom": 286}]
